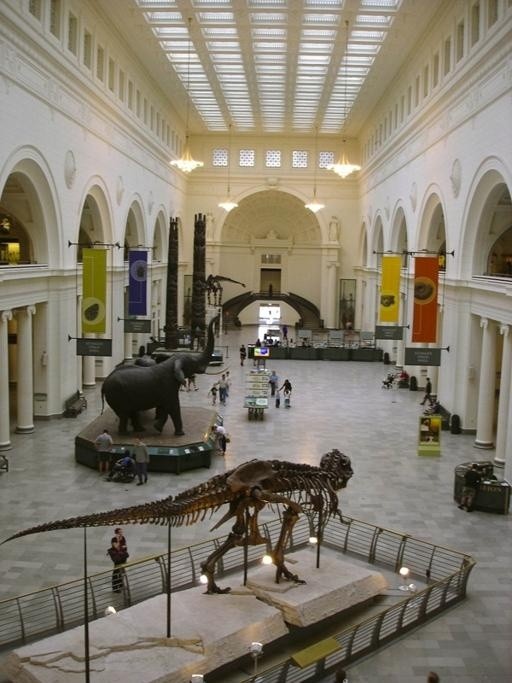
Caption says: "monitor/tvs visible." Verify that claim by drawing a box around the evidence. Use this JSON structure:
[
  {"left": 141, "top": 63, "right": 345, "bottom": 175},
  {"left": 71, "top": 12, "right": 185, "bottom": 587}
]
[{"left": 253, "top": 347, "right": 270, "bottom": 357}]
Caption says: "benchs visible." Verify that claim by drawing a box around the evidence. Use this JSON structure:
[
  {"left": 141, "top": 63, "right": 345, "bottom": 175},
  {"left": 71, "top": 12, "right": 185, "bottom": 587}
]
[
  {"left": 63, "top": 390, "right": 87, "bottom": 417},
  {"left": 428, "top": 403, "right": 451, "bottom": 428}
]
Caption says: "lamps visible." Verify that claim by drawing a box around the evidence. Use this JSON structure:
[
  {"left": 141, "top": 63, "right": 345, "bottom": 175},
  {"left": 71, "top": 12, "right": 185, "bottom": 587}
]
[
  {"left": 331, "top": 21, "right": 359, "bottom": 181},
  {"left": 170, "top": 17, "right": 205, "bottom": 174},
  {"left": 305, "top": 127, "right": 326, "bottom": 212},
  {"left": 221, "top": 121, "right": 240, "bottom": 211}
]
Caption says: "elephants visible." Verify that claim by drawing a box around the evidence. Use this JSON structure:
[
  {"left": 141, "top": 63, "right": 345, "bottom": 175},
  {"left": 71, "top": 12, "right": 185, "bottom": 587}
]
[{"left": 102, "top": 315, "right": 232, "bottom": 435}]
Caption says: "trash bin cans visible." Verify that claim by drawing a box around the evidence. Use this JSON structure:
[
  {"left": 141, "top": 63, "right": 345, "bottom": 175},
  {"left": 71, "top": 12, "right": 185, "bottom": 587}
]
[
  {"left": 384, "top": 352, "right": 389, "bottom": 364},
  {"left": 139, "top": 346, "right": 145, "bottom": 357},
  {"left": 410, "top": 376, "right": 417, "bottom": 391},
  {"left": 452, "top": 415, "right": 461, "bottom": 434}
]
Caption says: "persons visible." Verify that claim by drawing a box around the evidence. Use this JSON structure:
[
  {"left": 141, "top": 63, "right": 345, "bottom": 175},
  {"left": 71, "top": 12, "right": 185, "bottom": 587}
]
[
  {"left": 331, "top": 670, "right": 349, "bottom": 683},
  {"left": 422, "top": 400, "right": 440, "bottom": 415},
  {"left": 103, "top": 455, "right": 136, "bottom": 482},
  {"left": 419, "top": 376, "right": 434, "bottom": 405},
  {"left": 180, "top": 325, "right": 293, "bottom": 407},
  {"left": 129, "top": 437, "right": 150, "bottom": 485},
  {"left": 94, "top": 429, "right": 113, "bottom": 475},
  {"left": 426, "top": 670, "right": 439, "bottom": 683},
  {"left": 210, "top": 423, "right": 229, "bottom": 456},
  {"left": 111, "top": 527, "right": 129, "bottom": 592}
]
